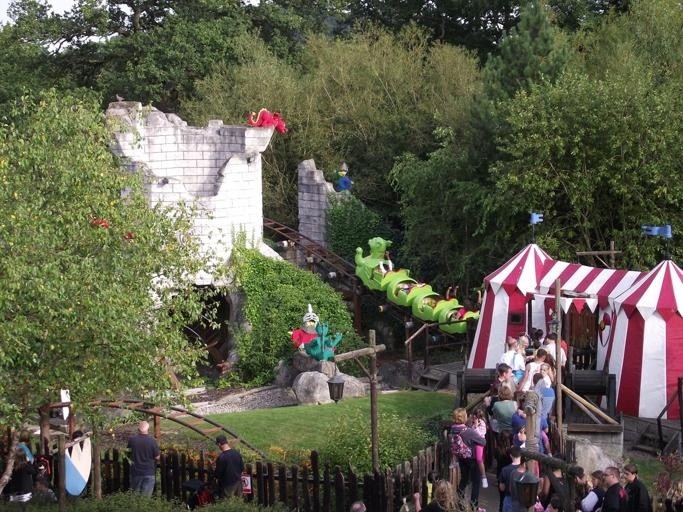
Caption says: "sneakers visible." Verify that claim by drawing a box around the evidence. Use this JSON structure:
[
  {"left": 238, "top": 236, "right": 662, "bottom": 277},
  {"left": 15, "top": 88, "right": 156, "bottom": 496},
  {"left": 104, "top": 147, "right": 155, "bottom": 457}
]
[
  {"left": 474, "top": 507, "right": 486, "bottom": 512},
  {"left": 481, "top": 476, "right": 489, "bottom": 489}
]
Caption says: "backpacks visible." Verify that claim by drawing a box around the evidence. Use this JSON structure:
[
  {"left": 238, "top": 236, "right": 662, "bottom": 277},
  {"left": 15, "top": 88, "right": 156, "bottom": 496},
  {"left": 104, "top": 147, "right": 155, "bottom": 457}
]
[{"left": 448, "top": 425, "right": 473, "bottom": 458}]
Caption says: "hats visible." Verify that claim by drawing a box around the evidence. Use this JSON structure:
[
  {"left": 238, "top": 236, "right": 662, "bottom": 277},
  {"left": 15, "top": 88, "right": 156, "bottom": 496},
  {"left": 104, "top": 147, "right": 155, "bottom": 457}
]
[{"left": 214, "top": 434, "right": 227, "bottom": 445}]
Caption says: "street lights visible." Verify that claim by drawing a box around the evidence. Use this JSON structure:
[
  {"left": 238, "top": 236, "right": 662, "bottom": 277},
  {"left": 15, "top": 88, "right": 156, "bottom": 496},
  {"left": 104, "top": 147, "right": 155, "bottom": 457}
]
[{"left": 328, "top": 347, "right": 381, "bottom": 475}]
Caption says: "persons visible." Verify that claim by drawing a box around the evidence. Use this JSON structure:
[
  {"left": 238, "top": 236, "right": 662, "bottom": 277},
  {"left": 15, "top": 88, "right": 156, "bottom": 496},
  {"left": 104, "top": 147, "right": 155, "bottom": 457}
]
[
  {"left": 666, "top": 479, "right": 683, "bottom": 511},
  {"left": 531, "top": 342, "right": 542, "bottom": 358},
  {"left": 497, "top": 428, "right": 515, "bottom": 472},
  {"left": 126, "top": 421, "right": 160, "bottom": 496},
  {"left": 11, "top": 448, "right": 41, "bottom": 505},
  {"left": 513, "top": 393, "right": 549, "bottom": 453},
  {"left": 496, "top": 337, "right": 526, "bottom": 383},
  {"left": 581, "top": 470, "right": 605, "bottom": 511},
  {"left": 475, "top": 290, "right": 485, "bottom": 311},
  {"left": 569, "top": 466, "right": 592, "bottom": 511},
  {"left": 215, "top": 434, "right": 245, "bottom": 499},
  {"left": 595, "top": 467, "right": 630, "bottom": 511},
  {"left": 414, "top": 479, "right": 476, "bottom": 511},
  {"left": 378, "top": 249, "right": 394, "bottom": 277},
  {"left": 523, "top": 326, "right": 544, "bottom": 342},
  {"left": 529, "top": 373, "right": 556, "bottom": 418},
  {"left": 16, "top": 430, "right": 36, "bottom": 463},
  {"left": 543, "top": 353, "right": 557, "bottom": 379},
  {"left": 68, "top": 430, "right": 88, "bottom": 496},
  {"left": 448, "top": 407, "right": 487, "bottom": 512},
  {"left": 624, "top": 463, "right": 653, "bottom": 511},
  {"left": 455, "top": 298, "right": 474, "bottom": 322},
  {"left": 531, "top": 363, "right": 552, "bottom": 390},
  {"left": 468, "top": 408, "right": 488, "bottom": 490},
  {"left": 548, "top": 493, "right": 568, "bottom": 511},
  {"left": 542, "top": 454, "right": 565, "bottom": 506},
  {"left": 509, "top": 455, "right": 528, "bottom": 503},
  {"left": 493, "top": 365, "right": 512, "bottom": 394},
  {"left": 492, "top": 386, "right": 518, "bottom": 431},
  {"left": 446, "top": 286, "right": 459, "bottom": 300},
  {"left": 517, "top": 349, "right": 547, "bottom": 392},
  {"left": 498, "top": 444, "right": 523, "bottom": 510},
  {"left": 656, "top": 470, "right": 673, "bottom": 512},
  {"left": 351, "top": 501, "right": 366, "bottom": 512},
  {"left": 542, "top": 335, "right": 568, "bottom": 369},
  {"left": 518, "top": 424, "right": 541, "bottom": 449}
]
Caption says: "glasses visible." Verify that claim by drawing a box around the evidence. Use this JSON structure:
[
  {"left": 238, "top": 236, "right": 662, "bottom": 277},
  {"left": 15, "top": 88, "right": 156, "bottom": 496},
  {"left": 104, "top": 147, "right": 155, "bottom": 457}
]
[{"left": 603, "top": 473, "right": 614, "bottom": 476}]
